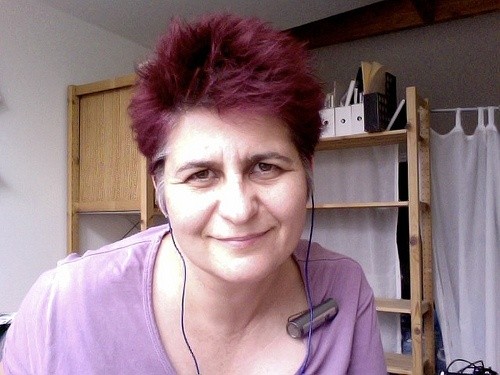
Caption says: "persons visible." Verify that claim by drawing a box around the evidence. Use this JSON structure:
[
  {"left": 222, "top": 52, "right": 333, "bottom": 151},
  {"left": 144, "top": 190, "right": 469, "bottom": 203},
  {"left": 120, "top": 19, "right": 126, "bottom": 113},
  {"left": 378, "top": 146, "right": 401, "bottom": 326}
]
[{"left": 0, "top": 11, "right": 388, "bottom": 375}]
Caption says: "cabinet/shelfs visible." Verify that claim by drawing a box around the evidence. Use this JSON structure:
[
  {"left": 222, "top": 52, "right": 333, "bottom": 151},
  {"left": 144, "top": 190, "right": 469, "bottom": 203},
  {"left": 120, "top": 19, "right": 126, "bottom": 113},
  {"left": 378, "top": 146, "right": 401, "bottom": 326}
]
[{"left": 308, "top": 86, "right": 434, "bottom": 375}]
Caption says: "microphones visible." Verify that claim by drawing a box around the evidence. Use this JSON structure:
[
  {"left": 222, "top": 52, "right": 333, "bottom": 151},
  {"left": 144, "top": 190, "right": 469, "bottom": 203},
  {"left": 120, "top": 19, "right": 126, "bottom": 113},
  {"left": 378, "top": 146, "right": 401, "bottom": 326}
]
[{"left": 286, "top": 298, "right": 339, "bottom": 339}]
